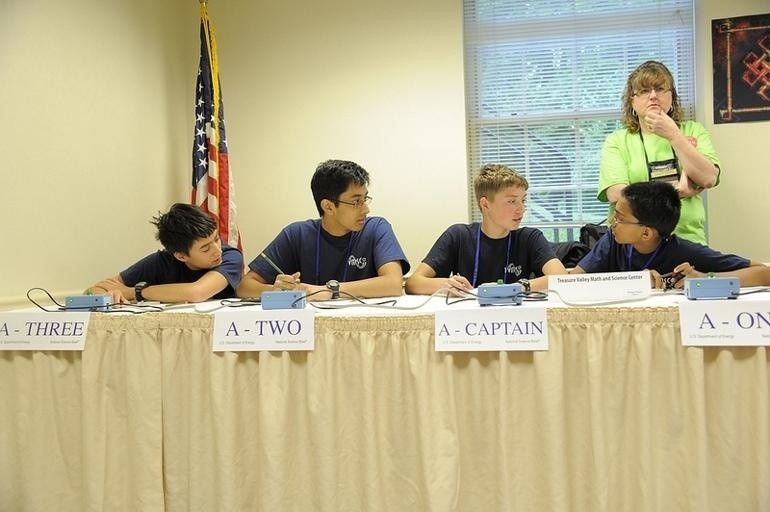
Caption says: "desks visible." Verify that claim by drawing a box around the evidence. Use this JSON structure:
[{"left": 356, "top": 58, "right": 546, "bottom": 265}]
[{"left": 1, "top": 283, "right": 770, "bottom": 511}]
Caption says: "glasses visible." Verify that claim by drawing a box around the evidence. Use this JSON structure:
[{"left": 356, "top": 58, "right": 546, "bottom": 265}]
[
  {"left": 328, "top": 195, "right": 373, "bottom": 209},
  {"left": 607, "top": 214, "right": 645, "bottom": 228},
  {"left": 630, "top": 84, "right": 672, "bottom": 100}
]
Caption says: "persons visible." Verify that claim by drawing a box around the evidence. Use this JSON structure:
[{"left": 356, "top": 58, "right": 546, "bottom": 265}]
[
  {"left": 400, "top": 162, "right": 571, "bottom": 299},
  {"left": 563, "top": 178, "right": 770, "bottom": 290},
  {"left": 79, "top": 201, "right": 246, "bottom": 305},
  {"left": 594, "top": 56, "right": 724, "bottom": 250},
  {"left": 232, "top": 156, "right": 415, "bottom": 303}
]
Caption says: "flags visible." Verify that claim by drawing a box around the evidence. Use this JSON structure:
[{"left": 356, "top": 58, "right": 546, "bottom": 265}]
[{"left": 187, "top": 0, "right": 245, "bottom": 260}]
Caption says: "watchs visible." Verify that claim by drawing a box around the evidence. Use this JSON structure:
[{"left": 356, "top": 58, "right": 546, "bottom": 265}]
[
  {"left": 326, "top": 278, "right": 341, "bottom": 300},
  {"left": 706, "top": 270, "right": 716, "bottom": 279},
  {"left": 518, "top": 277, "right": 531, "bottom": 295},
  {"left": 134, "top": 280, "right": 153, "bottom": 303}
]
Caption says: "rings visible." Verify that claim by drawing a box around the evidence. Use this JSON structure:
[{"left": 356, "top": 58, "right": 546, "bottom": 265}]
[{"left": 648, "top": 123, "right": 653, "bottom": 130}]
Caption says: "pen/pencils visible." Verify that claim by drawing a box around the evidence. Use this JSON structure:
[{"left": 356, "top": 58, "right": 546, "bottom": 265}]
[
  {"left": 457, "top": 272, "right": 466, "bottom": 288},
  {"left": 261, "top": 252, "right": 285, "bottom": 275}
]
